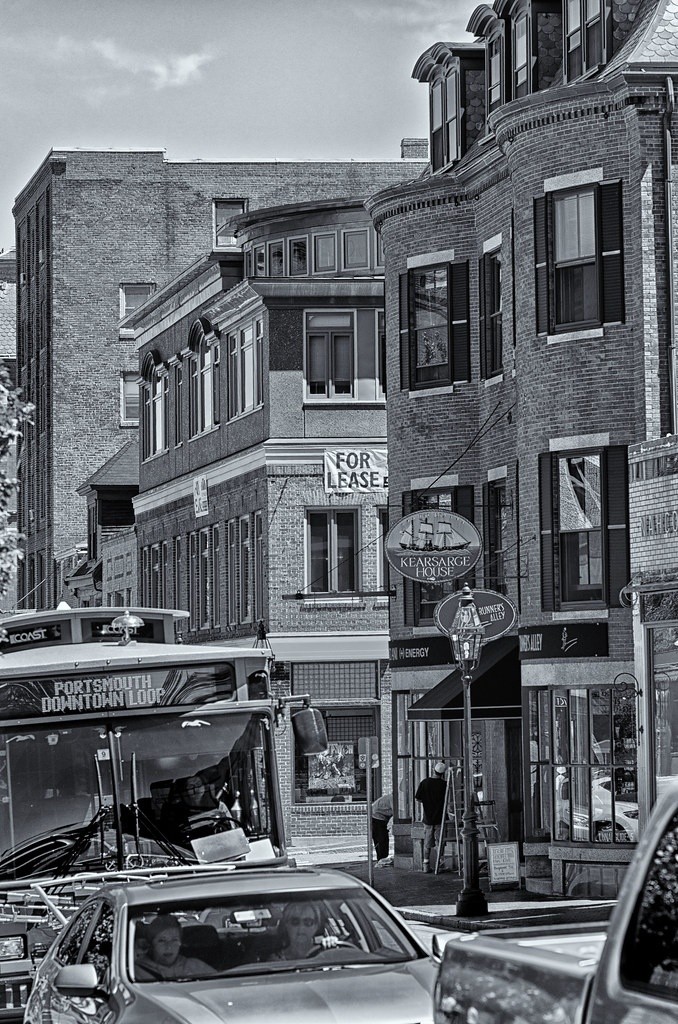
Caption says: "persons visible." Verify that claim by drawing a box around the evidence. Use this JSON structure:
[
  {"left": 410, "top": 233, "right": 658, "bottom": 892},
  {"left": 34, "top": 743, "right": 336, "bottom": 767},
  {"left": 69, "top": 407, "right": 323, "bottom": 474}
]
[
  {"left": 370, "top": 790, "right": 400, "bottom": 867},
  {"left": 134, "top": 916, "right": 219, "bottom": 981},
  {"left": 272, "top": 902, "right": 343, "bottom": 962},
  {"left": 161, "top": 770, "right": 237, "bottom": 850},
  {"left": 414, "top": 763, "right": 452, "bottom": 873}
]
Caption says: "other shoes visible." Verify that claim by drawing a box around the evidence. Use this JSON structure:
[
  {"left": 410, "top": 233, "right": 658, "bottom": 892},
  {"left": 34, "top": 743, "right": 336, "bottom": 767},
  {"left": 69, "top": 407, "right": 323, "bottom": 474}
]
[
  {"left": 423, "top": 862, "right": 432, "bottom": 873},
  {"left": 438, "top": 861, "right": 446, "bottom": 870}
]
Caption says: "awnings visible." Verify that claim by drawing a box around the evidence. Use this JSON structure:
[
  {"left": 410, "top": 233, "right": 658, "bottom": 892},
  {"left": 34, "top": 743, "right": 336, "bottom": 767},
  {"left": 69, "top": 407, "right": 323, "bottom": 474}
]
[{"left": 406, "top": 637, "right": 521, "bottom": 721}]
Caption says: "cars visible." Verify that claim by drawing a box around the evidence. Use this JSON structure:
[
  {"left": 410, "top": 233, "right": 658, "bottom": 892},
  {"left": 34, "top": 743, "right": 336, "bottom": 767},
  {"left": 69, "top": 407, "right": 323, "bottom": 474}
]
[
  {"left": 548, "top": 767, "right": 640, "bottom": 846},
  {"left": 25, "top": 864, "right": 444, "bottom": 1024}
]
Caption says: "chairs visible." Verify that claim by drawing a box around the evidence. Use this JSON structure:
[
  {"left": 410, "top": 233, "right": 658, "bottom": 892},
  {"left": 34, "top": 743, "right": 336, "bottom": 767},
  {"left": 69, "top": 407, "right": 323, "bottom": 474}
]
[{"left": 246, "top": 930, "right": 279, "bottom": 964}]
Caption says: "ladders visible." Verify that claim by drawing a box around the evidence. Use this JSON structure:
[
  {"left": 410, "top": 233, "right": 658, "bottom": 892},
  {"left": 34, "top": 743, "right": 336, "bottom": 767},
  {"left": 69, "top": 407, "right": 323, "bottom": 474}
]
[{"left": 434, "top": 767, "right": 489, "bottom": 873}]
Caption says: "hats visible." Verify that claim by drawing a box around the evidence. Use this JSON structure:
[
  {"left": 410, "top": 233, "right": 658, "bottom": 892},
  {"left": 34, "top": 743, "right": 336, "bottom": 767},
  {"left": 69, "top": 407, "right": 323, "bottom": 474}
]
[{"left": 434, "top": 763, "right": 446, "bottom": 776}]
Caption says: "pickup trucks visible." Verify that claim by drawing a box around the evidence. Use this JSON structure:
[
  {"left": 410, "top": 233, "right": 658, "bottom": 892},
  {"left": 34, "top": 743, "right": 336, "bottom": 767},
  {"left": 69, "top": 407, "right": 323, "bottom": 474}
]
[{"left": 430, "top": 782, "right": 678, "bottom": 1024}]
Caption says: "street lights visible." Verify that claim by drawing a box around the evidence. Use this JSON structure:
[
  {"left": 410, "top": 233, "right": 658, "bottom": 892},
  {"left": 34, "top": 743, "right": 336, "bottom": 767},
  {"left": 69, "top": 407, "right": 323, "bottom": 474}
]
[
  {"left": 4, "top": 735, "right": 21, "bottom": 852},
  {"left": 450, "top": 581, "right": 488, "bottom": 918}
]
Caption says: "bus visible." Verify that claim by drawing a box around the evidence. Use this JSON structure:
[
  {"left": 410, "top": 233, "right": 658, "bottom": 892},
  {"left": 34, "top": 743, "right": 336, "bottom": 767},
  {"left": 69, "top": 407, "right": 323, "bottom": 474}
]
[{"left": 1, "top": 603, "right": 330, "bottom": 1024}]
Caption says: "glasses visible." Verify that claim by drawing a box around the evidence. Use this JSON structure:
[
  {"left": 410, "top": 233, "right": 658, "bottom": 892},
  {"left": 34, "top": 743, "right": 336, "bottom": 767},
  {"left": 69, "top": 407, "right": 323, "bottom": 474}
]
[
  {"left": 186, "top": 781, "right": 202, "bottom": 789},
  {"left": 288, "top": 917, "right": 317, "bottom": 927}
]
